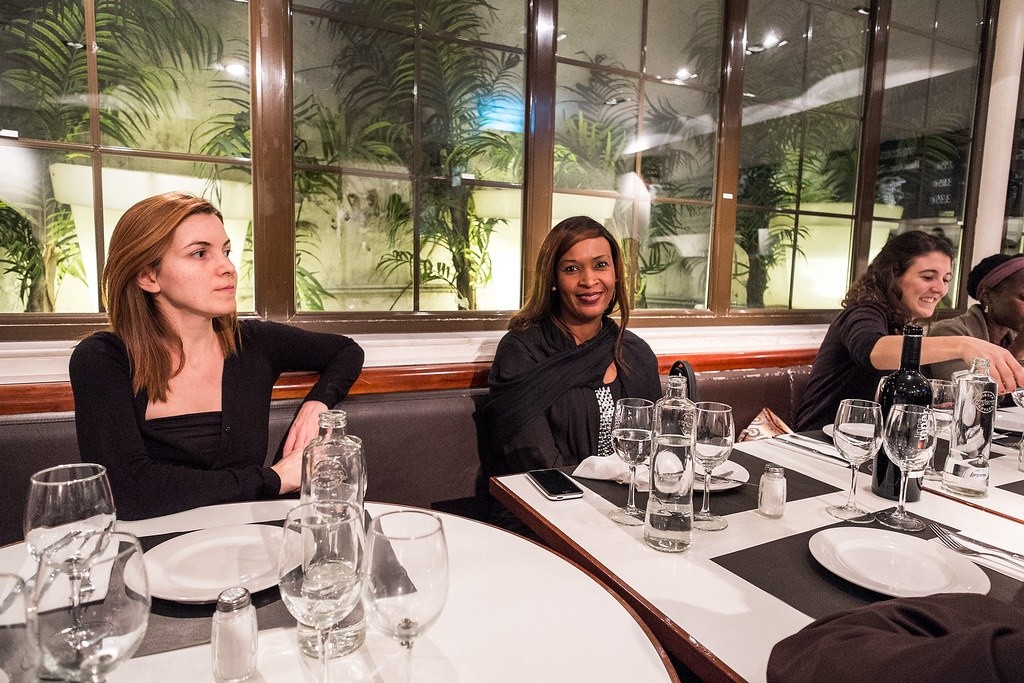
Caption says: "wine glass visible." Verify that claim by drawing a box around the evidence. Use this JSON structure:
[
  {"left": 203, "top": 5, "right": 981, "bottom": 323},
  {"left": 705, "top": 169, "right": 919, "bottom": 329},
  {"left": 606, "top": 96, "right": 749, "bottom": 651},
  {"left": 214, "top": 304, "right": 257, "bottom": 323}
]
[
  {"left": 30, "top": 532, "right": 151, "bottom": 683},
  {"left": 883, "top": 403, "right": 937, "bottom": 531},
  {"left": 276, "top": 499, "right": 368, "bottom": 682},
  {"left": 26, "top": 460, "right": 116, "bottom": 659},
  {"left": 825, "top": 398, "right": 884, "bottom": 524},
  {"left": 690, "top": 401, "right": 736, "bottom": 531},
  {"left": 611, "top": 397, "right": 656, "bottom": 524},
  {"left": 922, "top": 379, "right": 958, "bottom": 481},
  {"left": 359, "top": 510, "right": 450, "bottom": 682}
]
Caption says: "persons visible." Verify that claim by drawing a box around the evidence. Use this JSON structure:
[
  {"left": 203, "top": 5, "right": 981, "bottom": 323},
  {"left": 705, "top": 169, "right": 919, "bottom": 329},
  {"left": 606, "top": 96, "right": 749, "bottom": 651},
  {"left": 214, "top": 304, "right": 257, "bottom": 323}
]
[
  {"left": 65, "top": 192, "right": 364, "bottom": 523},
  {"left": 483, "top": 216, "right": 663, "bottom": 474},
  {"left": 796, "top": 232, "right": 1024, "bottom": 433},
  {"left": 764, "top": 582, "right": 1024, "bottom": 683},
  {"left": 926, "top": 251, "right": 1024, "bottom": 383}
]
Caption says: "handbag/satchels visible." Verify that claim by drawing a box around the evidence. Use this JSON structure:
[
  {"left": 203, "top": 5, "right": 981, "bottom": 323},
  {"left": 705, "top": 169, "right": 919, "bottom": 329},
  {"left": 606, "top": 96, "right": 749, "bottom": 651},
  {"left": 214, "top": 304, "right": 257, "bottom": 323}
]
[{"left": 736, "top": 409, "right": 794, "bottom": 444}]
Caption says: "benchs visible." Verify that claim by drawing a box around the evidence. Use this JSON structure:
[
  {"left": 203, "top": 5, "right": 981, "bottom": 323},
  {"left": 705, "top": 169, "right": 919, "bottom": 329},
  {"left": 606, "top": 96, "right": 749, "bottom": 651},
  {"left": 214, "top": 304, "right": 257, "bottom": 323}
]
[{"left": 0, "top": 364, "right": 814, "bottom": 550}]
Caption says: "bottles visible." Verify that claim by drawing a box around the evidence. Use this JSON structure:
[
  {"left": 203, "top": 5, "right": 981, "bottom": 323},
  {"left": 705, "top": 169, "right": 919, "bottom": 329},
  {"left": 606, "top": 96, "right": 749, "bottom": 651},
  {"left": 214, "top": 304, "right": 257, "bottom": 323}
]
[
  {"left": 940, "top": 357, "right": 998, "bottom": 497},
  {"left": 1012, "top": 388, "right": 1024, "bottom": 409},
  {"left": 298, "top": 410, "right": 367, "bottom": 657},
  {"left": 1017, "top": 432, "right": 1024, "bottom": 473},
  {"left": 757, "top": 462, "right": 787, "bottom": 517},
  {"left": 871, "top": 325, "right": 933, "bottom": 502},
  {"left": 211, "top": 587, "right": 259, "bottom": 683},
  {"left": 644, "top": 376, "right": 697, "bottom": 553}
]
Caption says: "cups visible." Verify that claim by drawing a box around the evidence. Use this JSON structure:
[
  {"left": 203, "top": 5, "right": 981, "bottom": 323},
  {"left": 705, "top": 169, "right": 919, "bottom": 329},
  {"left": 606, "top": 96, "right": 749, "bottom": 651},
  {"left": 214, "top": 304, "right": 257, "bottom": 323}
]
[{"left": 0, "top": 573, "right": 40, "bottom": 683}]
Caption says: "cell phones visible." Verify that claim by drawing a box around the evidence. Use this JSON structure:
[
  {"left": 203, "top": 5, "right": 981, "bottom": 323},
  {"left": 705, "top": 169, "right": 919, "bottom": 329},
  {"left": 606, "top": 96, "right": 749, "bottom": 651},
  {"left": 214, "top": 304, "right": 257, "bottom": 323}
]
[{"left": 526, "top": 468, "right": 584, "bottom": 500}]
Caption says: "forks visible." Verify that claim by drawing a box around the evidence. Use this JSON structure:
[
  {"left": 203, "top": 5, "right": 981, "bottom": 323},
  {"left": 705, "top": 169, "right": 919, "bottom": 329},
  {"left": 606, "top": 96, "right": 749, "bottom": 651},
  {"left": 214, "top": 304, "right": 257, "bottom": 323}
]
[
  {"left": 695, "top": 471, "right": 733, "bottom": 483},
  {"left": 929, "top": 523, "right": 1024, "bottom": 570},
  {"left": 66, "top": 521, "right": 114, "bottom": 618}
]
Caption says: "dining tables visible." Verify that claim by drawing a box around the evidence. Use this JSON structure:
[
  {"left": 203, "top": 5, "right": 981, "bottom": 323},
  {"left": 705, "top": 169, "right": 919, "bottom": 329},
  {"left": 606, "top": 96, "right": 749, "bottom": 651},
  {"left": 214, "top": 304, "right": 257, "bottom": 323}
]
[{"left": 0, "top": 394, "right": 1024, "bottom": 683}]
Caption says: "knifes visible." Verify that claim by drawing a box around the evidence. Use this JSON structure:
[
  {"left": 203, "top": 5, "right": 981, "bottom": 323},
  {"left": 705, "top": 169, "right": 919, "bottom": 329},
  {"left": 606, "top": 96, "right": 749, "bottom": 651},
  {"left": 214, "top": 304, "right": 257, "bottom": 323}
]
[
  {"left": 773, "top": 437, "right": 849, "bottom": 464},
  {"left": 695, "top": 472, "right": 757, "bottom": 488},
  {"left": 942, "top": 528, "right": 1024, "bottom": 560},
  {"left": 996, "top": 408, "right": 1017, "bottom": 414}
]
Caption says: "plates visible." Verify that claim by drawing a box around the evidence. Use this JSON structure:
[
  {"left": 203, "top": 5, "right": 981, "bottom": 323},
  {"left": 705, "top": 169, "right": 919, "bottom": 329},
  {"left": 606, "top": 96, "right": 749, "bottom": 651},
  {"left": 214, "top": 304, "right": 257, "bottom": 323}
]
[
  {"left": 643, "top": 455, "right": 749, "bottom": 490},
  {"left": 994, "top": 412, "right": 1024, "bottom": 432},
  {"left": 123, "top": 524, "right": 306, "bottom": 604},
  {"left": 823, "top": 423, "right": 875, "bottom": 439},
  {"left": 808, "top": 527, "right": 991, "bottom": 596}
]
